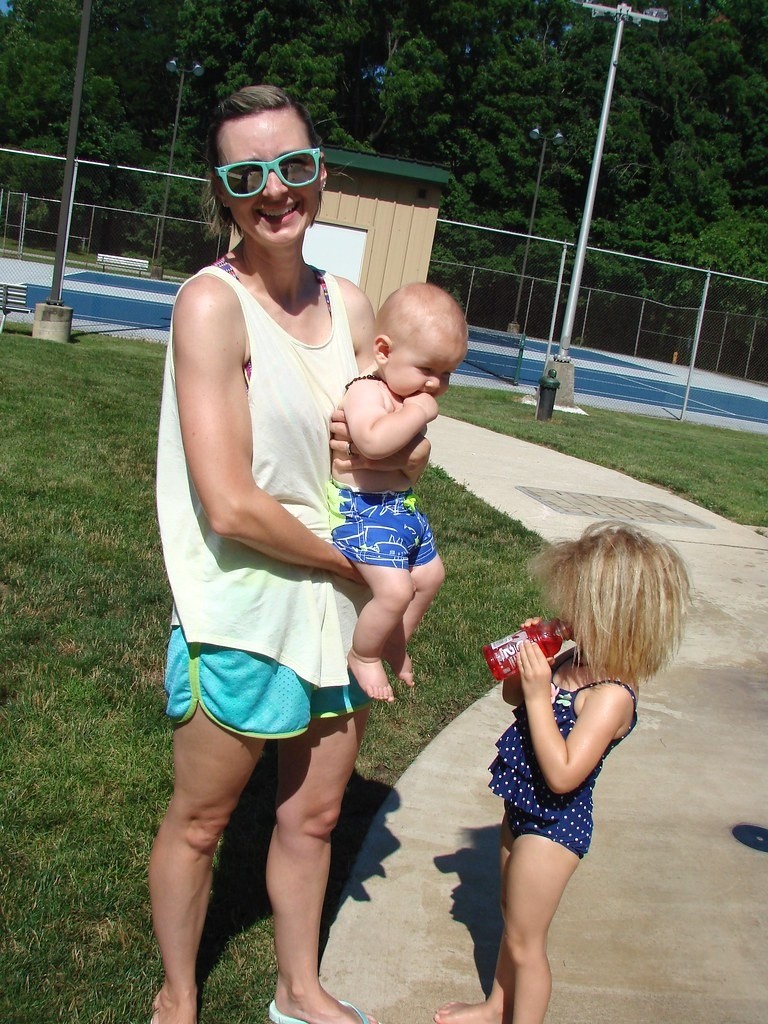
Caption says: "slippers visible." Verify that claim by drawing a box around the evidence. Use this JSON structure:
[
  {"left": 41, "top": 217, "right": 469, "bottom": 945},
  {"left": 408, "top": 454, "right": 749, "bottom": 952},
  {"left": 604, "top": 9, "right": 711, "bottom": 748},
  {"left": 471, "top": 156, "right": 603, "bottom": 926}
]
[{"left": 268, "top": 993, "right": 382, "bottom": 1024}]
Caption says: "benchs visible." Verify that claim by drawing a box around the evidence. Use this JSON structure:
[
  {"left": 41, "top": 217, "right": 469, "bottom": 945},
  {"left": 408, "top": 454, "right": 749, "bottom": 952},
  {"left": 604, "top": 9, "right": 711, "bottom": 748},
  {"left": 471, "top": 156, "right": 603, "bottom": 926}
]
[
  {"left": 95, "top": 254, "right": 149, "bottom": 277},
  {"left": 0, "top": 280, "right": 35, "bottom": 335}
]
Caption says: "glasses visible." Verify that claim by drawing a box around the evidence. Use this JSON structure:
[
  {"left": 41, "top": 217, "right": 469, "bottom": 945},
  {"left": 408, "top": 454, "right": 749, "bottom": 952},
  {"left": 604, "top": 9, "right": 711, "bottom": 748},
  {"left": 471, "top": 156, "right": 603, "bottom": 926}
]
[{"left": 213, "top": 147, "right": 322, "bottom": 200}]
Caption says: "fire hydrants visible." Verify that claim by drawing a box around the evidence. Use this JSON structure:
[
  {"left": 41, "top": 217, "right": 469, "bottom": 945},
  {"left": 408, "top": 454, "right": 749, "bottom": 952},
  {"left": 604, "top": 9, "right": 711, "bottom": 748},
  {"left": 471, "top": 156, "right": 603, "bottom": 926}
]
[{"left": 536, "top": 369, "right": 560, "bottom": 422}]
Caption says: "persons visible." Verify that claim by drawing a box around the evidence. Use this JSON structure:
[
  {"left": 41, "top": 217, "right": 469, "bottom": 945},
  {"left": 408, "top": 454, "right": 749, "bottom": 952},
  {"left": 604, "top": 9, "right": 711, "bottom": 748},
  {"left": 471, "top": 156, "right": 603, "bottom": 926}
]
[
  {"left": 325, "top": 282, "right": 469, "bottom": 701},
  {"left": 434, "top": 520, "right": 690, "bottom": 1024},
  {"left": 150, "top": 84, "right": 431, "bottom": 1024}
]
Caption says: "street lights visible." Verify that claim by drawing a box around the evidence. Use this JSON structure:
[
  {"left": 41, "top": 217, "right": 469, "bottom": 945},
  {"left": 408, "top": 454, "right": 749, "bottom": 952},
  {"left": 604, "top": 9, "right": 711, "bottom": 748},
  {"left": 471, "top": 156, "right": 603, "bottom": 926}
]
[
  {"left": 506, "top": 127, "right": 564, "bottom": 334},
  {"left": 149, "top": 59, "right": 204, "bottom": 281},
  {"left": 536, "top": 0, "right": 668, "bottom": 407}
]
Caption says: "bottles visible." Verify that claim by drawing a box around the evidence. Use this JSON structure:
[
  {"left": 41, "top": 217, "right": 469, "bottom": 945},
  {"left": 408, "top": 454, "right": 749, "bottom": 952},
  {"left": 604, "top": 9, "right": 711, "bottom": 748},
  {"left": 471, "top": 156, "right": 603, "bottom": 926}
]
[{"left": 483, "top": 618, "right": 572, "bottom": 681}]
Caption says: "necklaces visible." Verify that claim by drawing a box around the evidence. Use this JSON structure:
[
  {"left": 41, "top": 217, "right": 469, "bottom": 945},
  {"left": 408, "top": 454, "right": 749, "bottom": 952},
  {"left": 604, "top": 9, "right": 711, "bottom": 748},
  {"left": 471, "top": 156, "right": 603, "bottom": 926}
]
[
  {"left": 572, "top": 650, "right": 588, "bottom": 667},
  {"left": 345, "top": 375, "right": 382, "bottom": 390}
]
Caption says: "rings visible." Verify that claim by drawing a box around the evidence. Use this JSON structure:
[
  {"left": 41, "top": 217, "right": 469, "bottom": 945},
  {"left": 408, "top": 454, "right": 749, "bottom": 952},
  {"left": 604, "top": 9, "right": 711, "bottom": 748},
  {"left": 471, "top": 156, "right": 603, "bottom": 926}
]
[{"left": 346, "top": 440, "right": 354, "bottom": 456}]
